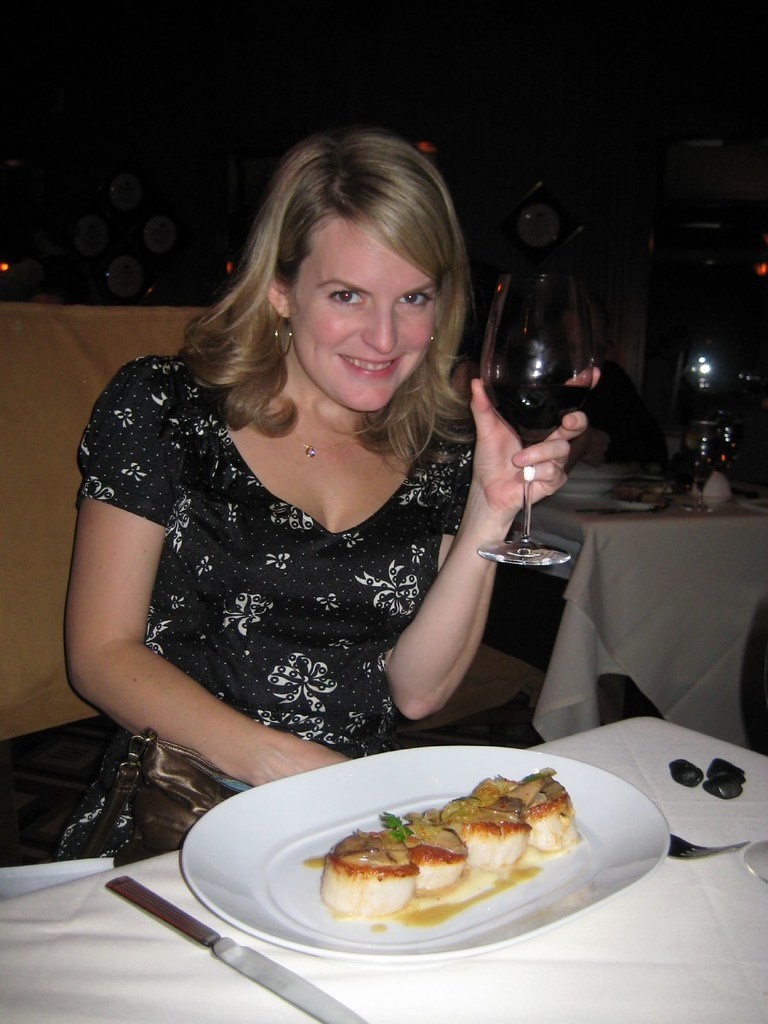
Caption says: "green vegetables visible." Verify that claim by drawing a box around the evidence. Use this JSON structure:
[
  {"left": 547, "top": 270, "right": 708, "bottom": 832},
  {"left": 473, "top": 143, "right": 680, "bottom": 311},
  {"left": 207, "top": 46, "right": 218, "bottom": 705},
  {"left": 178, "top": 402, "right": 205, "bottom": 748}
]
[{"left": 383, "top": 811, "right": 415, "bottom": 843}]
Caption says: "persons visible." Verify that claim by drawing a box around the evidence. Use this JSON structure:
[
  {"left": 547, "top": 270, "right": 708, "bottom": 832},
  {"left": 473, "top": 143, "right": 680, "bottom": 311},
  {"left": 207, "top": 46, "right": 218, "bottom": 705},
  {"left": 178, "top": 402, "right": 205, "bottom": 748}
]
[
  {"left": 56, "top": 126, "right": 601, "bottom": 868},
  {"left": 498, "top": 290, "right": 671, "bottom": 472}
]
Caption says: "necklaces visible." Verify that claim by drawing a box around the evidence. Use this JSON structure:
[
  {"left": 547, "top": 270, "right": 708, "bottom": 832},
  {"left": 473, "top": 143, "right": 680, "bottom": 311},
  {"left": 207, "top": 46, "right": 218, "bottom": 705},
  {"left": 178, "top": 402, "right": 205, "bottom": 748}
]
[{"left": 284, "top": 427, "right": 362, "bottom": 459}]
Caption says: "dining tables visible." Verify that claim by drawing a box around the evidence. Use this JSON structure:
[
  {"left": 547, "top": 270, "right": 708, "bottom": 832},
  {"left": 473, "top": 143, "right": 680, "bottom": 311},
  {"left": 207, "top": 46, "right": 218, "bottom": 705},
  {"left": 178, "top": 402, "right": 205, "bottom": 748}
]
[
  {"left": 0, "top": 717, "right": 768, "bottom": 1024},
  {"left": 505, "top": 472, "right": 768, "bottom": 751}
]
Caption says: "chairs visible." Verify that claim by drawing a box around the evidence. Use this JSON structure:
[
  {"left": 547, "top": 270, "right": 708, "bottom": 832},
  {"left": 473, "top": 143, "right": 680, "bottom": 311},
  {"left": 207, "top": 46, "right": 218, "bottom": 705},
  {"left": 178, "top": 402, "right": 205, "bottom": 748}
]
[
  {"left": 393, "top": 641, "right": 548, "bottom": 750},
  {"left": 0, "top": 300, "right": 221, "bottom": 742}
]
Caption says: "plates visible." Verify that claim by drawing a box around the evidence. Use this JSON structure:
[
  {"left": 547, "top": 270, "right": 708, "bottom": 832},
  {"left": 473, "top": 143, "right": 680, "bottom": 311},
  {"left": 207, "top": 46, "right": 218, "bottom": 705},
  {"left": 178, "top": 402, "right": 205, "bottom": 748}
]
[
  {"left": 608, "top": 493, "right": 669, "bottom": 509},
  {"left": 180, "top": 746, "right": 670, "bottom": 964},
  {"left": 559, "top": 481, "right": 612, "bottom": 495}
]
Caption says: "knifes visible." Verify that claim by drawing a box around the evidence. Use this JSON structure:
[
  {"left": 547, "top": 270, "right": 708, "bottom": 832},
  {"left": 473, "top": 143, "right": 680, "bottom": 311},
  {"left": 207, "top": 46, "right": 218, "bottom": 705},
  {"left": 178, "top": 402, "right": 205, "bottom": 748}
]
[{"left": 102, "top": 874, "right": 366, "bottom": 1024}]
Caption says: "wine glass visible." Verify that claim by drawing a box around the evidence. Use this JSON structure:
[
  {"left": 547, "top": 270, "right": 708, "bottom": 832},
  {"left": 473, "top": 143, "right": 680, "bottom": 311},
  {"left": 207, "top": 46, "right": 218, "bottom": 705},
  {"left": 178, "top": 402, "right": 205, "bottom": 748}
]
[
  {"left": 678, "top": 409, "right": 744, "bottom": 512},
  {"left": 480, "top": 272, "right": 596, "bottom": 565}
]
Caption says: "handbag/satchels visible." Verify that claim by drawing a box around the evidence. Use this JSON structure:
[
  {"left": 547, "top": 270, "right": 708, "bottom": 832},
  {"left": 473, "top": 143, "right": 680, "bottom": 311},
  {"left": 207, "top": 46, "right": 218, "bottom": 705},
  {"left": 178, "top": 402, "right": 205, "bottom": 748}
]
[{"left": 80, "top": 729, "right": 242, "bottom": 868}]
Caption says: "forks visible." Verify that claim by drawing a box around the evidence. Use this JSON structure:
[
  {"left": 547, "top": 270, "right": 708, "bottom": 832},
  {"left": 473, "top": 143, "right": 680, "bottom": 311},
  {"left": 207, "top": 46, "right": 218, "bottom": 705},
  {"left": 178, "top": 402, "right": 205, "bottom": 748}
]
[{"left": 669, "top": 835, "right": 751, "bottom": 860}]
